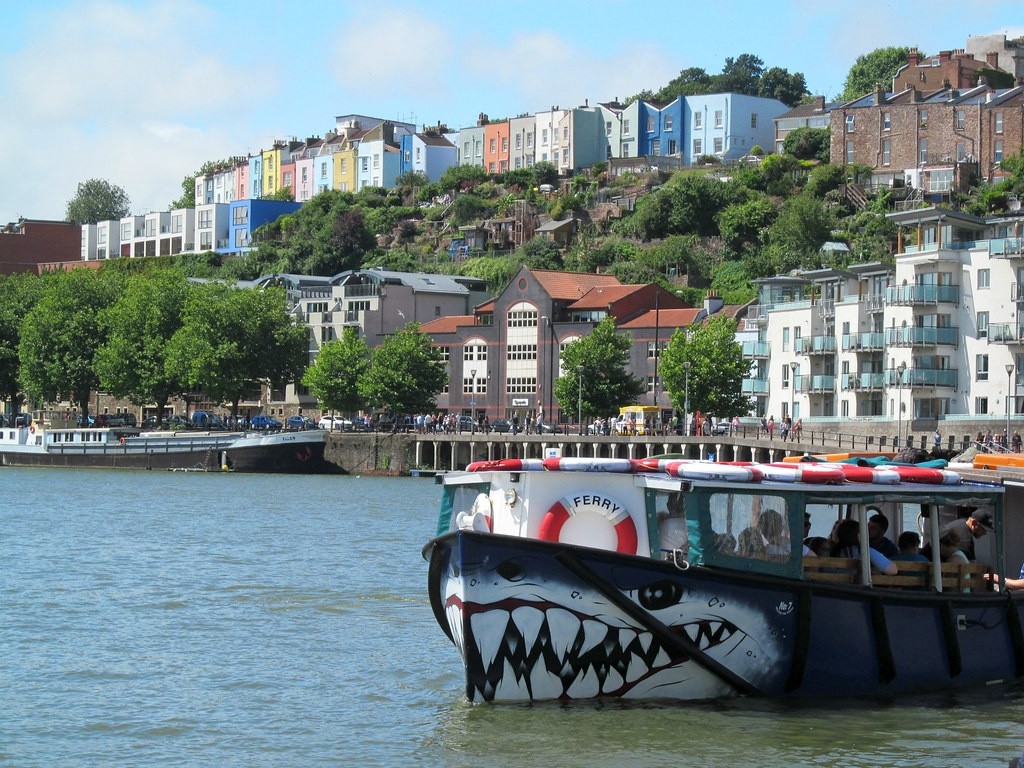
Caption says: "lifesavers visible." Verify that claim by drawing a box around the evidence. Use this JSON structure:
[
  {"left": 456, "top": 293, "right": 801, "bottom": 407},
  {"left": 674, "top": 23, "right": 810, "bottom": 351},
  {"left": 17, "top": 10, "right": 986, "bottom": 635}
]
[
  {"left": 754, "top": 462, "right": 901, "bottom": 485},
  {"left": 537, "top": 489, "right": 639, "bottom": 556},
  {"left": 30, "top": 426, "right": 35, "bottom": 433},
  {"left": 120, "top": 437, "right": 124, "bottom": 445},
  {"left": 465, "top": 457, "right": 545, "bottom": 471},
  {"left": 544, "top": 456, "right": 639, "bottom": 473},
  {"left": 875, "top": 463, "right": 961, "bottom": 485},
  {"left": 635, "top": 458, "right": 763, "bottom": 483},
  {"left": 472, "top": 492, "right": 492, "bottom": 529}
]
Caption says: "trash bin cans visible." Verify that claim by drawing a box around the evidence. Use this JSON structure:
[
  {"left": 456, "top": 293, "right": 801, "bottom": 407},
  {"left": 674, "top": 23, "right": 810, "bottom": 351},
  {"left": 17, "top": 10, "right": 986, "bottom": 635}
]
[
  {"left": 603, "top": 428, "right": 610, "bottom": 436},
  {"left": 241, "top": 420, "right": 249, "bottom": 430}
]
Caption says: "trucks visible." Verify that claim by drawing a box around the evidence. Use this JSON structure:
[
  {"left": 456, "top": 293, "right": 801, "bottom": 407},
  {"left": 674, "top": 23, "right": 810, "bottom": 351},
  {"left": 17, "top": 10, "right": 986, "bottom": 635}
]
[{"left": 611, "top": 406, "right": 660, "bottom": 435}]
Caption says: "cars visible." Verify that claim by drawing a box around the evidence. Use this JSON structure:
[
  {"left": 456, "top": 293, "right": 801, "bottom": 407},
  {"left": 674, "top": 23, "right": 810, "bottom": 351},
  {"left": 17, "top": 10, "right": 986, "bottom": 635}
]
[
  {"left": 287, "top": 416, "right": 315, "bottom": 429},
  {"left": 193, "top": 411, "right": 221, "bottom": 423},
  {"left": 165, "top": 415, "right": 192, "bottom": 425},
  {"left": 523, "top": 421, "right": 562, "bottom": 433},
  {"left": 141, "top": 415, "right": 168, "bottom": 425},
  {"left": 491, "top": 419, "right": 510, "bottom": 432},
  {"left": 460, "top": 416, "right": 479, "bottom": 431},
  {"left": 318, "top": 415, "right": 353, "bottom": 430},
  {"left": 352, "top": 418, "right": 364, "bottom": 431},
  {"left": 711, "top": 422, "right": 736, "bottom": 435},
  {"left": 78, "top": 413, "right": 137, "bottom": 427},
  {"left": 250, "top": 416, "right": 283, "bottom": 429}
]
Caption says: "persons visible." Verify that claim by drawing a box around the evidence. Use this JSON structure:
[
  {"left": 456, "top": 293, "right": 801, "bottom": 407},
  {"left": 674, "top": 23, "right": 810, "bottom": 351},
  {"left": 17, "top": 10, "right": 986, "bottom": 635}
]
[
  {"left": 208, "top": 413, "right": 232, "bottom": 430},
  {"left": 780, "top": 415, "right": 802, "bottom": 439},
  {"left": 644, "top": 417, "right": 657, "bottom": 436},
  {"left": 760, "top": 415, "right": 774, "bottom": 438},
  {"left": 303, "top": 415, "right": 310, "bottom": 431},
  {"left": 975, "top": 428, "right": 1007, "bottom": 450},
  {"left": 594, "top": 415, "right": 636, "bottom": 436},
  {"left": 732, "top": 416, "right": 740, "bottom": 436},
  {"left": 513, "top": 413, "right": 542, "bottom": 434},
  {"left": 691, "top": 416, "right": 712, "bottom": 436},
  {"left": 934, "top": 430, "right": 941, "bottom": 447},
  {"left": 1012, "top": 431, "right": 1021, "bottom": 453},
  {"left": 364, "top": 412, "right": 461, "bottom": 434},
  {"left": 668, "top": 415, "right": 678, "bottom": 430},
  {"left": 657, "top": 492, "right": 1024, "bottom": 590},
  {"left": 477, "top": 415, "right": 488, "bottom": 433}
]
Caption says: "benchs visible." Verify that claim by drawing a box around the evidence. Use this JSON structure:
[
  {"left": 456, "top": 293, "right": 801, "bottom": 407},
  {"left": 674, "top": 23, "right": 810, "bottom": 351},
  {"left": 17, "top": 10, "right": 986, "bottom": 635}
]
[{"left": 765, "top": 555, "right": 995, "bottom": 593}]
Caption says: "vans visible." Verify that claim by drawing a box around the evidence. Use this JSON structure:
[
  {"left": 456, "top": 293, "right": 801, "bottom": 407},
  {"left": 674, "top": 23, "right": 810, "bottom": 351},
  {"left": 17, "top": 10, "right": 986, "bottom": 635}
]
[{"left": 371, "top": 412, "right": 392, "bottom": 431}]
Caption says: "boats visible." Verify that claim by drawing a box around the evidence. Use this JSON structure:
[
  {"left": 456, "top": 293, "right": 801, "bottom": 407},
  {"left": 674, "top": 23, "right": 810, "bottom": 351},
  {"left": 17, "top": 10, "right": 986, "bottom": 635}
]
[
  {"left": 0, "top": 426, "right": 330, "bottom": 473},
  {"left": 420, "top": 450, "right": 1024, "bottom": 712}
]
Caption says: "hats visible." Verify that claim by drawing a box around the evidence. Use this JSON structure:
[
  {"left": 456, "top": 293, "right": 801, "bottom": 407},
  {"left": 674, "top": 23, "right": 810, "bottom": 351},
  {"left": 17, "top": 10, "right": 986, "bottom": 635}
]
[{"left": 973, "top": 509, "right": 995, "bottom": 531}]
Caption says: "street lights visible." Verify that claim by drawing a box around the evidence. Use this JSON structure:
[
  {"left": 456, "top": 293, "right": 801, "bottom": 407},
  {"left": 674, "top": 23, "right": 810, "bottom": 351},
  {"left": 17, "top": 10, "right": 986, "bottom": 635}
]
[
  {"left": 1005, "top": 365, "right": 1015, "bottom": 443},
  {"left": 684, "top": 362, "right": 690, "bottom": 435},
  {"left": 790, "top": 362, "right": 797, "bottom": 422},
  {"left": 897, "top": 366, "right": 904, "bottom": 449},
  {"left": 576, "top": 365, "right": 583, "bottom": 434},
  {"left": 471, "top": 370, "right": 476, "bottom": 418}
]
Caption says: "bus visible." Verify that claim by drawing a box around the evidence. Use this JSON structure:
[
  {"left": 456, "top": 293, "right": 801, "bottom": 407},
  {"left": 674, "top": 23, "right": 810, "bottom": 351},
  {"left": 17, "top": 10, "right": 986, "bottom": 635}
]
[{"left": 31, "top": 408, "right": 79, "bottom": 430}]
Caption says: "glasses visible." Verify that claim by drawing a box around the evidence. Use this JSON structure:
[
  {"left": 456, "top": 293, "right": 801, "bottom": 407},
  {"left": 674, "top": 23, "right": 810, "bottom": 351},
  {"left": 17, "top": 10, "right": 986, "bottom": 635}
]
[{"left": 804, "top": 522, "right": 812, "bottom": 527}]
